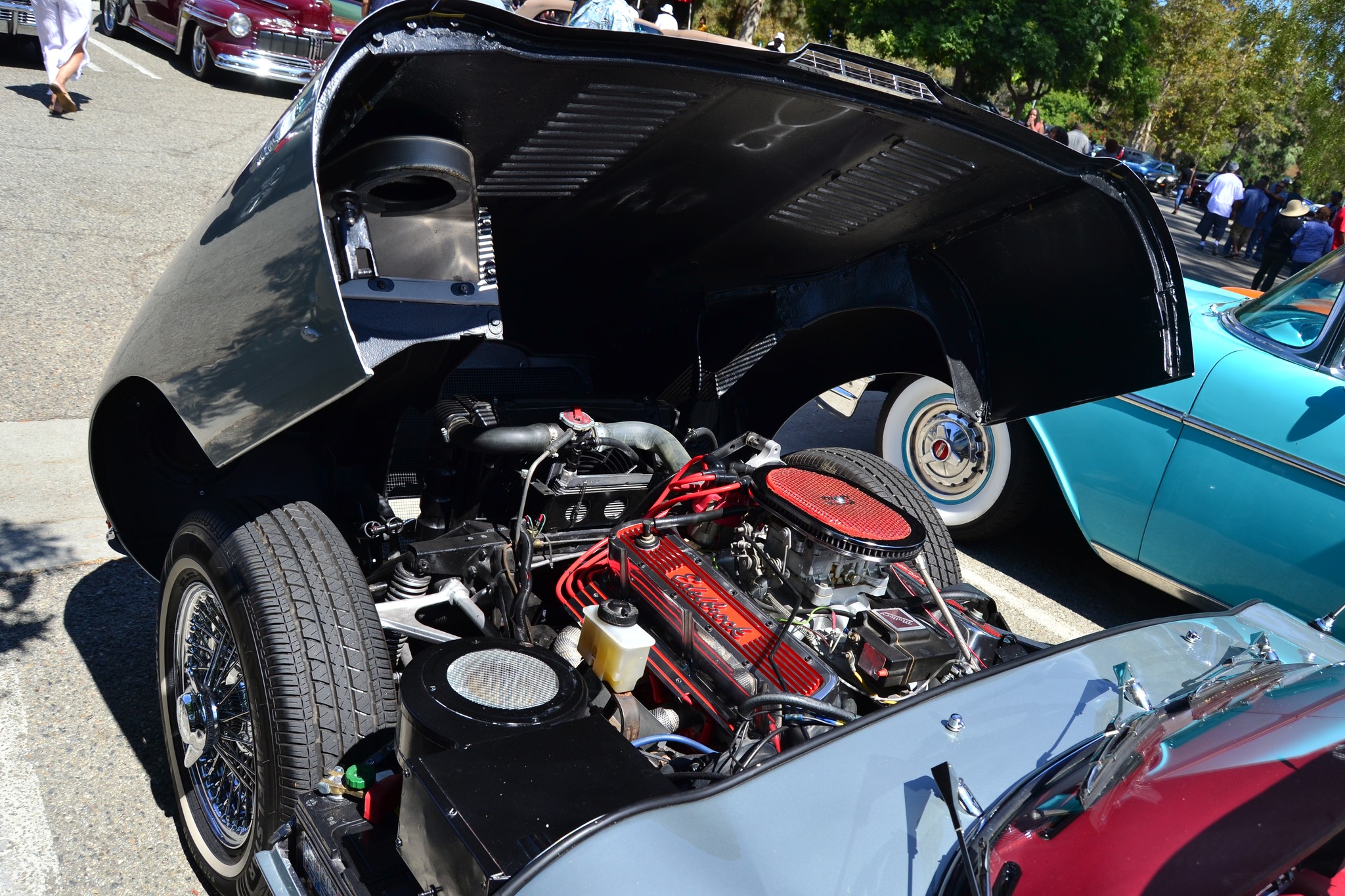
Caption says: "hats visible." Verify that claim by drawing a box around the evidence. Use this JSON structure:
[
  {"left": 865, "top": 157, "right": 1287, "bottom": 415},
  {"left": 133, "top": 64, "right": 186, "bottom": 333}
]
[
  {"left": 774, "top": 32, "right": 786, "bottom": 41},
  {"left": 1279, "top": 199, "right": 1310, "bottom": 216},
  {"left": 1228, "top": 162, "right": 1239, "bottom": 170},
  {"left": 660, "top": 4, "right": 674, "bottom": 15}
]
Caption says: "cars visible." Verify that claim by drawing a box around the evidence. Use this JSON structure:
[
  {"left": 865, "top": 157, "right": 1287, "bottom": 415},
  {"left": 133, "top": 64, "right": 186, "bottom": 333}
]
[
  {"left": 816, "top": 245, "right": 1345, "bottom": 644},
  {"left": 88, "top": 0, "right": 1345, "bottom": 896},
  {"left": 1020, "top": 121, "right": 1244, "bottom": 207},
  {"left": 99, "top": 0, "right": 360, "bottom": 88}
]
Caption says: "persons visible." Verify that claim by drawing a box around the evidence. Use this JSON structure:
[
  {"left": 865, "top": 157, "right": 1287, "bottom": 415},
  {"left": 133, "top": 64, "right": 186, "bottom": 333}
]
[
  {"left": 696, "top": 15, "right": 707, "bottom": 32},
  {"left": 568, "top": 0, "right": 636, "bottom": 32},
  {"left": 30, "top": 0, "right": 92, "bottom": 115},
  {"left": 1194, "top": 161, "right": 1345, "bottom": 293},
  {"left": 1171, "top": 161, "right": 1197, "bottom": 215},
  {"left": 361, "top": 0, "right": 401, "bottom": 19},
  {"left": 764, "top": 32, "right": 786, "bottom": 53},
  {"left": 624, "top": 0, "right": 679, "bottom": 35},
  {"left": 1094, "top": 139, "right": 1119, "bottom": 159},
  {"left": 1066, "top": 123, "right": 1093, "bottom": 157},
  {"left": 1022, "top": 108, "right": 1069, "bottom": 147}
]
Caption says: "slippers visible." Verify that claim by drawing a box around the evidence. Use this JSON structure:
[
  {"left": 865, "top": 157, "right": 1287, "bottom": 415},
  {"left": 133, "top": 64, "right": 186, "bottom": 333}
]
[{"left": 49, "top": 80, "right": 77, "bottom": 115}]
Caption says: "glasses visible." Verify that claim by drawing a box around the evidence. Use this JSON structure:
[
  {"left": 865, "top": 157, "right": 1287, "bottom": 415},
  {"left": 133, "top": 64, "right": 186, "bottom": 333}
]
[{"left": 1277, "top": 184, "right": 1283, "bottom": 189}]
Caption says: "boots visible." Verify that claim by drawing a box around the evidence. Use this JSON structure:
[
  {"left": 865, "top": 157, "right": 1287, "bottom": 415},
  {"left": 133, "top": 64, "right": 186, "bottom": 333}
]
[{"left": 1172, "top": 204, "right": 1180, "bottom": 214}]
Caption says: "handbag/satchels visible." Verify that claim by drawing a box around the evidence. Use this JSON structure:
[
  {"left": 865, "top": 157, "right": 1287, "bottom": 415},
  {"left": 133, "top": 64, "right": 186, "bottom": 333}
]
[{"left": 1184, "top": 187, "right": 1193, "bottom": 197}]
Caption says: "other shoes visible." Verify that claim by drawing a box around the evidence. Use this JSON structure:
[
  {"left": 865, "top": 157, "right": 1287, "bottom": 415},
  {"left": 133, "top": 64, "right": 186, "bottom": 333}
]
[
  {"left": 1196, "top": 244, "right": 1204, "bottom": 251},
  {"left": 1225, "top": 252, "right": 1239, "bottom": 258},
  {"left": 1212, "top": 244, "right": 1218, "bottom": 256}
]
